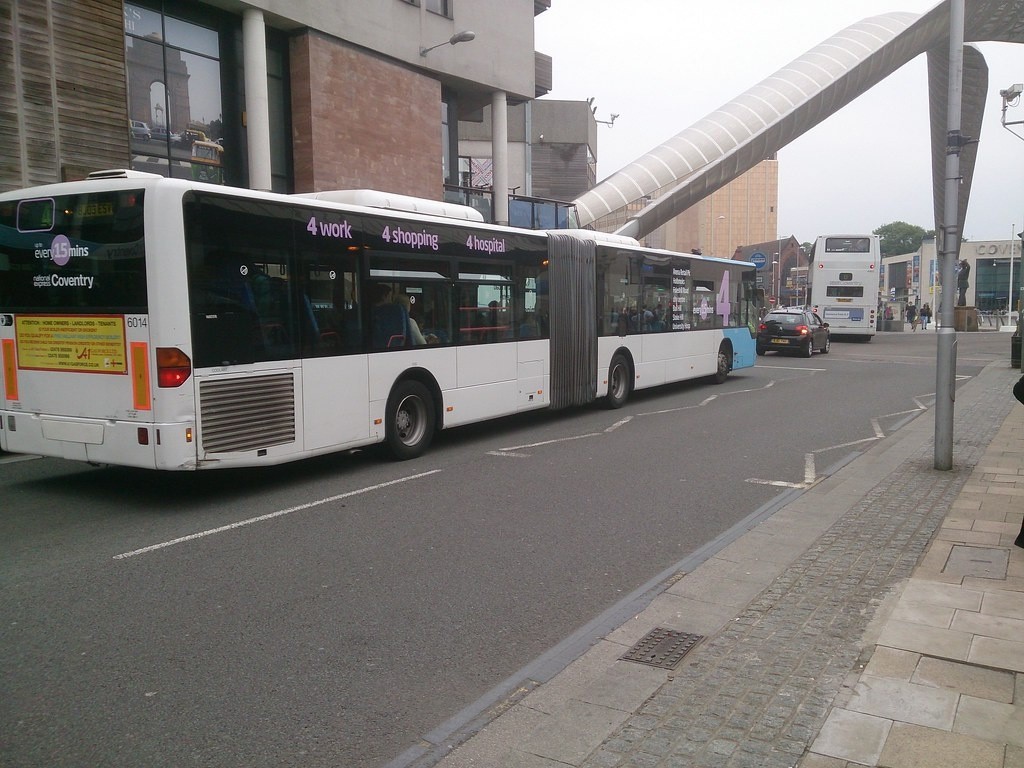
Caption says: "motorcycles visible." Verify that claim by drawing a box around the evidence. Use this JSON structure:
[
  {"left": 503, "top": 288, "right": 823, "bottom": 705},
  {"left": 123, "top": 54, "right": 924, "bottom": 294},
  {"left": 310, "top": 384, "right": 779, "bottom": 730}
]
[
  {"left": 190, "top": 139, "right": 225, "bottom": 185},
  {"left": 184, "top": 129, "right": 211, "bottom": 147}
]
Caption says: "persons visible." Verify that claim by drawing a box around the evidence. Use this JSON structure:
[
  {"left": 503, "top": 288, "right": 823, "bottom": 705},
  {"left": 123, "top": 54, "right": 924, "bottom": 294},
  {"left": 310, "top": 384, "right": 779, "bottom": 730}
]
[
  {"left": 616, "top": 301, "right": 671, "bottom": 332},
  {"left": 486, "top": 299, "right": 513, "bottom": 328},
  {"left": 878, "top": 301, "right": 893, "bottom": 320},
  {"left": 368, "top": 284, "right": 440, "bottom": 346},
  {"left": 905, "top": 301, "right": 932, "bottom": 332},
  {"left": 956, "top": 259, "right": 970, "bottom": 306}
]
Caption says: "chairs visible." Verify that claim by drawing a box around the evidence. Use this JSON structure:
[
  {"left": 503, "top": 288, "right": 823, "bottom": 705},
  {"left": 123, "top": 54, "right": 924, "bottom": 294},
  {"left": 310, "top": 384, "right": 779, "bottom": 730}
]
[
  {"left": 300, "top": 288, "right": 343, "bottom": 355},
  {"left": 351, "top": 303, "right": 407, "bottom": 353},
  {"left": 778, "top": 314, "right": 788, "bottom": 323},
  {"left": 400, "top": 304, "right": 413, "bottom": 346},
  {"left": 794, "top": 316, "right": 803, "bottom": 324}
]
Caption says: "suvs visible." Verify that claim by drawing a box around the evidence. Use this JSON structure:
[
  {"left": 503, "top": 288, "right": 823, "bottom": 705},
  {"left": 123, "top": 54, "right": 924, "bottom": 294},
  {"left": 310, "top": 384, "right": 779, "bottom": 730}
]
[{"left": 130, "top": 120, "right": 152, "bottom": 141}]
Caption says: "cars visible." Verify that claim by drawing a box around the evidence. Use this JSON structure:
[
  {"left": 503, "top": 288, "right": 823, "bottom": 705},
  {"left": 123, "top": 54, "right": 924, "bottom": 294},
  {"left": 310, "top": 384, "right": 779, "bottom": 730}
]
[
  {"left": 757, "top": 306, "right": 832, "bottom": 357},
  {"left": 150, "top": 127, "right": 181, "bottom": 142}
]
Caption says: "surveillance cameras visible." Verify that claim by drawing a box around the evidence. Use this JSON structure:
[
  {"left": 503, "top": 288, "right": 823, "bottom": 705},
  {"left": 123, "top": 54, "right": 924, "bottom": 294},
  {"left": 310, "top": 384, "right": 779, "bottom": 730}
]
[
  {"left": 610, "top": 112, "right": 619, "bottom": 118},
  {"left": 540, "top": 135, "right": 543, "bottom": 139},
  {"left": 1006, "top": 84, "right": 1024, "bottom": 102}
]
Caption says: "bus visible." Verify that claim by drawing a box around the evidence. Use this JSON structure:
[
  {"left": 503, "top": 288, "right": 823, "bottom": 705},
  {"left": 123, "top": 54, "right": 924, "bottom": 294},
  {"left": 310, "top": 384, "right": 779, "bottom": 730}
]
[
  {"left": 0, "top": 168, "right": 758, "bottom": 473},
  {"left": 805, "top": 233, "right": 881, "bottom": 342}
]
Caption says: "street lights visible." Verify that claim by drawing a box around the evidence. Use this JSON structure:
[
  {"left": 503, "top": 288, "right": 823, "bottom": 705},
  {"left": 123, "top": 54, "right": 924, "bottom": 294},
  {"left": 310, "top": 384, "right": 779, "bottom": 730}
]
[
  {"left": 475, "top": 183, "right": 489, "bottom": 195},
  {"left": 508, "top": 186, "right": 521, "bottom": 200},
  {"left": 714, "top": 216, "right": 725, "bottom": 257},
  {"left": 771, "top": 252, "right": 781, "bottom": 308},
  {"left": 776, "top": 234, "right": 789, "bottom": 307},
  {"left": 795, "top": 245, "right": 806, "bottom": 306}
]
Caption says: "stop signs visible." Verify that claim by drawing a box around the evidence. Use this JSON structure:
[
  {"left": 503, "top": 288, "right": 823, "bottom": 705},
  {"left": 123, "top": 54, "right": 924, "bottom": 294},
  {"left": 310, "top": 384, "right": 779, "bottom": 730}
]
[{"left": 769, "top": 297, "right": 775, "bottom": 305}]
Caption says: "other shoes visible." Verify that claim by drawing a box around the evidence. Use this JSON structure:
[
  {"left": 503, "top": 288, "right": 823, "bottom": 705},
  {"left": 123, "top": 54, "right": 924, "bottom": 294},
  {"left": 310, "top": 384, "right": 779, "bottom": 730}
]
[
  {"left": 925, "top": 327, "right": 927, "bottom": 330},
  {"left": 922, "top": 328, "right": 923, "bottom": 330}
]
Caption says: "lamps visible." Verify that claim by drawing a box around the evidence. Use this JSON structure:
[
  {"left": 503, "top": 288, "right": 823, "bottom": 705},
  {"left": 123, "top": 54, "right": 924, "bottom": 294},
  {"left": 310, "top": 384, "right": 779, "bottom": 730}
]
[
  {"left": 589, "top": 97, "right": 598, "bottom": 114},
  {"left": 992, "top": 259, "right": 996, "bottom": 267},
  {"left": 420, "top": 30, "right": 475, "bottom": 57}
]
